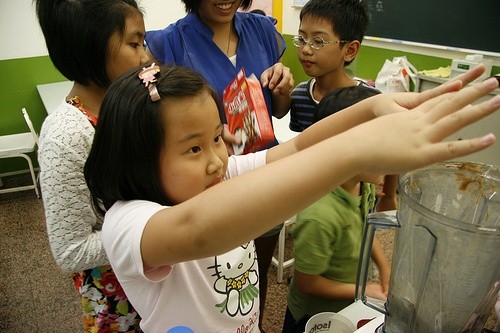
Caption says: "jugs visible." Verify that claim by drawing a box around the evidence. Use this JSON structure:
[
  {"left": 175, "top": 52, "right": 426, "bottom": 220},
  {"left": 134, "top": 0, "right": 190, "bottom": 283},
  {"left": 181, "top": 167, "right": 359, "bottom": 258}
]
[{"left": 355, "top": 161, "right": 500, "bottom": 333}]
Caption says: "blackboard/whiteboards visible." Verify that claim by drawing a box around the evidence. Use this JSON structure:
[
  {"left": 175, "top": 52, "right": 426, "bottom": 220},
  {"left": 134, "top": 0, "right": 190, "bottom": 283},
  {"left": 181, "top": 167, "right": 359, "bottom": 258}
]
[{"left": 361, "top": 0, "right": 500, "bottom": 58}]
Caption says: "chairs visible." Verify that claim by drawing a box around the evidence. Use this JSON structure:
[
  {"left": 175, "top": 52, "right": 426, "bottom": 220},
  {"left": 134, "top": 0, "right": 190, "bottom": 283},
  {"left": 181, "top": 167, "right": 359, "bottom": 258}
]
[{"left": 0, "top": 132, "right": 41, "bottom": 198}]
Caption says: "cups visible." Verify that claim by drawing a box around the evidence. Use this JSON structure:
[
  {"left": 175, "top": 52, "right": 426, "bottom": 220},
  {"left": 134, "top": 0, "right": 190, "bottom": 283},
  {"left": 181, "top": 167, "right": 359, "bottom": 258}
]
[{"left": 305, "top": 312, "right": 356, "bottom": 333}]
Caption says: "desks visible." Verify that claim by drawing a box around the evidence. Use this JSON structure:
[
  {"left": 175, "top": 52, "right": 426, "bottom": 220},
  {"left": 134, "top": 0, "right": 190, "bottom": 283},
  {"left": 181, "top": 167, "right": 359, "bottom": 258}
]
[{"left": 37, "top": 81, "right": 75, "bottom": 115}]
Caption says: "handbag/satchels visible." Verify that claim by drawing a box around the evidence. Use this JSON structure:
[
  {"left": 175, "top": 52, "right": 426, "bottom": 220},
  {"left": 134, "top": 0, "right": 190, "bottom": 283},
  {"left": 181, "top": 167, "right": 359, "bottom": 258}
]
[{"left": 374, "top": 57, "right": 419, "bottom": 94}]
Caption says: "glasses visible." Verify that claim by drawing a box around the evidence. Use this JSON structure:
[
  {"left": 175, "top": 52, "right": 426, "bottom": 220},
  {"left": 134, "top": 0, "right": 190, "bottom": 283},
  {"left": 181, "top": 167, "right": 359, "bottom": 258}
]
[{"left": 291, "top": 35, "right": 350, "bottom": 50}]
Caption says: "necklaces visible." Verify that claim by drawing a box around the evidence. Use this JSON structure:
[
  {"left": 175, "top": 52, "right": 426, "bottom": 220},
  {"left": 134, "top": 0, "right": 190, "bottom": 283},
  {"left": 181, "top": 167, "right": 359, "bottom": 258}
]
[{"left": 226, "top": 23, "right": 231, "bottom": 56}]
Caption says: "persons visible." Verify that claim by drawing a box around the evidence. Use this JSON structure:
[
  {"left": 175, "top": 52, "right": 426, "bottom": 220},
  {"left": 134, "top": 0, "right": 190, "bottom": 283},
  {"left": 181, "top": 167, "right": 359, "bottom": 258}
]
[
  {"left": 282, "top": 86, "right": 391, "bottom": 333},
  {"left": 83, "top": 64, "right": 500, "bottom": 333},
  {"left": 35, "top": 1, "right": 150, "bottom": 333},
  {"left": 143, "top": 1, "right": 294, "bottom": 333},
  {"left": 288, "top": 0, "right": 382, "bottom": 132}
]
